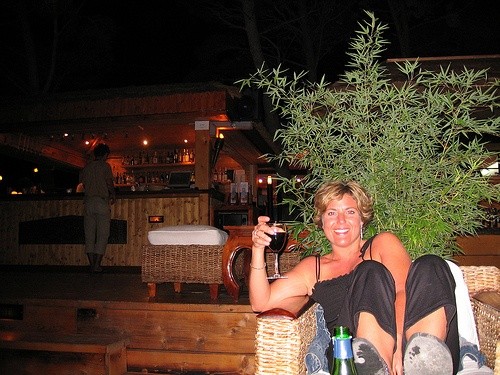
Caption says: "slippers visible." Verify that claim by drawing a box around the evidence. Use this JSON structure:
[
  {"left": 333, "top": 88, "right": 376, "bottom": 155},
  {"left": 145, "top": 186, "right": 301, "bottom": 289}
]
[
  {"left": 94, "top": 267, "right": 110, "bottom": 273},
  {"left": 87, "top": 269, "right": 93, "bottom": 273}
]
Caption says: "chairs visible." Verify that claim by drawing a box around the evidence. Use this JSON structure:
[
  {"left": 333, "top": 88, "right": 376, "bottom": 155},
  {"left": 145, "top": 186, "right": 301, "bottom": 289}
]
[{"left": 254, "top": 234, "right": 500, "bottom": 375}]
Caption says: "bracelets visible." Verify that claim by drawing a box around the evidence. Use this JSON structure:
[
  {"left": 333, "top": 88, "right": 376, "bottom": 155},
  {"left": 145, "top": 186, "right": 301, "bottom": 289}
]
[{"left": 250, "top": 262, "right": 267, "bottom": 269}]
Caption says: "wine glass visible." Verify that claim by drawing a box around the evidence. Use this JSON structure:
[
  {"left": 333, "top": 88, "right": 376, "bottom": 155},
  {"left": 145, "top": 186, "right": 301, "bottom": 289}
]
[{"left": 263, "top": 221, "right": 288, "bottom": 279}]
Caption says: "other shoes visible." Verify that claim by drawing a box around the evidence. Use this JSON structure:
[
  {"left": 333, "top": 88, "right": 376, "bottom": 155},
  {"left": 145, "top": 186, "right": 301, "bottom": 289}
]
[
  {"left": 351, "top": 338, "right": 391, "bottom": 375},
  {"left": 403, "top": 332, "right": 453, "bottom": 375}
]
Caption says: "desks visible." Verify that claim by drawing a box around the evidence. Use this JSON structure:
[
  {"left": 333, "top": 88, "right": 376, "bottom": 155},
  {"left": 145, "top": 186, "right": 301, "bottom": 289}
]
[
  {"left": 216, "top": 201, "right": 257, "bottom": 228},
  {"left": 222, "top": 225, "right": 318, "bottom": 304}
]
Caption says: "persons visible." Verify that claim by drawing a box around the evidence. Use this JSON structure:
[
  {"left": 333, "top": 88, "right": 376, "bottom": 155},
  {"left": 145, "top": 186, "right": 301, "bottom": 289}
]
[
  {"left": 80, "top": 144, "right": 133, "bottom": 271},
  {"left": 248, "top": 180, "right": 460, "bottom": 375}
]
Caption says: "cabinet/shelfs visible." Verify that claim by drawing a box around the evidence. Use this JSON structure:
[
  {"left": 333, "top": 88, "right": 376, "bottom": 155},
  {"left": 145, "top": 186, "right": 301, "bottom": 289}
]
[{"left": 115, "top": 162, "right": 195, "bottom": 186}]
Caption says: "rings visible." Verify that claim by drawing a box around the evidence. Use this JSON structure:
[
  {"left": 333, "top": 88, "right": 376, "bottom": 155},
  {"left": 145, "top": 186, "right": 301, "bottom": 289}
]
[{"left": 254, "top": 229, "right": 258, "bottom": 236}]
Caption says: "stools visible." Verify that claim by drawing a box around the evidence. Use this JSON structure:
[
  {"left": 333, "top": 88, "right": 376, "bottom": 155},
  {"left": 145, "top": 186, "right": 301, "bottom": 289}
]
[{"left": 140, "top": 223, "right": 245, "bottom": 302}]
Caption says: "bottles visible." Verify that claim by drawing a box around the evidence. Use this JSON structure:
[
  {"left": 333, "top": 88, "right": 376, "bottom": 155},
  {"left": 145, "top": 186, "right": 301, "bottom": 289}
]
[
  {"left": 116, "top": 149, "right": 194, "bottom": 186},
  {"left": 331, "top": 326, "right": 359, "bottom": 375},
  {"left": 213, "top": 168, "right": 227, "bottom": 181}
]
